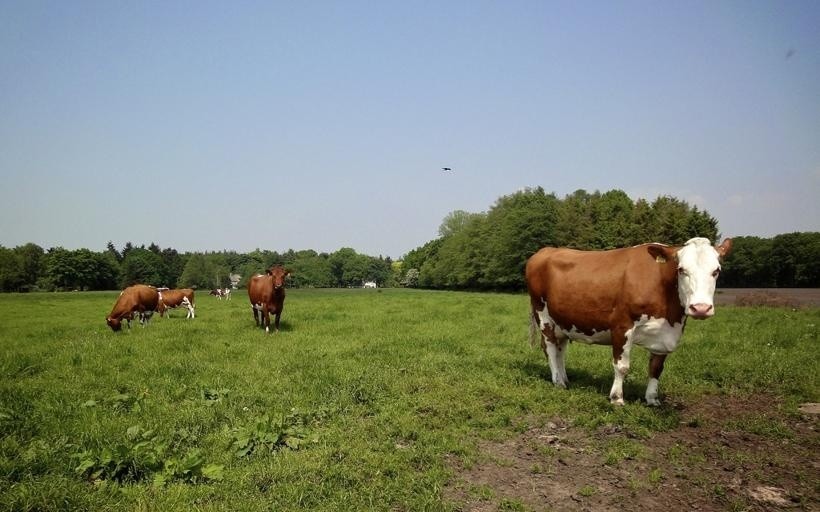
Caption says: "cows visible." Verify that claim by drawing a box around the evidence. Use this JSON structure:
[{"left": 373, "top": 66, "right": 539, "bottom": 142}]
[
  {"left": 523, "top": 233, "right": 732, "bottom": 406},
  {"left": 246, "top": 267, "right": 292, "bottom": 333},
  {"left": 208, "top": 288, "right": 232, "bottom": 300},
  {"left": 104, "top": 282, "right": 196, "bottom": 333}
]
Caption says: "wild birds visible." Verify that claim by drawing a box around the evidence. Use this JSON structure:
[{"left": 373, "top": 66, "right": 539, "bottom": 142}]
[{"left": 442, "top": 168, "right": 452, "bottom": 172}]
